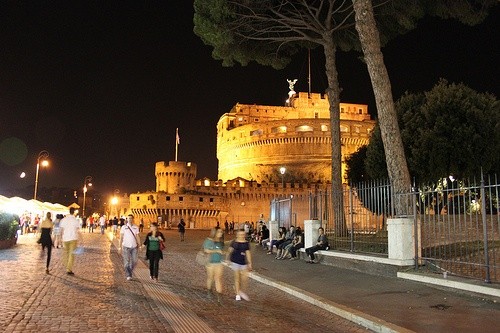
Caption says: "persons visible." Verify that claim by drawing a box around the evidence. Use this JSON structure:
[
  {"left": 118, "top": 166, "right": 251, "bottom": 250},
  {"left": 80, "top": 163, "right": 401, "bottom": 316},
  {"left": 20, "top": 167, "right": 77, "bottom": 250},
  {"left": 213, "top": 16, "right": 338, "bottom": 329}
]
[
  {"left": 244, "top": 219, "right": 304, "bottom": 260},
  {"left": 17, "top": 213, "right": 41, "bottom": 235},
  {"left": 304, "top": 226, "right": 328, "bottom": 264},
  {"left": 224, "top": 219, "right": 240, "bottom": 234},
  {"left": 202, "top": 225, "right": 227, "bottom": 302},
  {"left": 226, "top": 228, "right": 253, "bottom": 301},
  {"left": 178, "top": 218, "right": 186, "bottom": 241},
  {"left": 59, "top": 207, "right": 82, "bottom": 275},
  {"left": 20, "top": 211, "right": 66, "bottom": 247},
  {"left": 76, "top": 211, "right": 144, "bottom": 237},
  {"left": 39, "top": 211, "right": 53, "bottom": 275},
  {"left": 142, "top": 221, "right": 166, "bottom": 281},
  {"left": 118, "top": 213, "right": 142, "bottom": 280}
]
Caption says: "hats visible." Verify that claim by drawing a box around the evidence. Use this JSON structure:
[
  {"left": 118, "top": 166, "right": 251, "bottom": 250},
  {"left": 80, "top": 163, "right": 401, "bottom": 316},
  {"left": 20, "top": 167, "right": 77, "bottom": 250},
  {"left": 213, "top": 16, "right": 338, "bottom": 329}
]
[{"left": 259, "top": 221, "right": 263, "bottom": 223}]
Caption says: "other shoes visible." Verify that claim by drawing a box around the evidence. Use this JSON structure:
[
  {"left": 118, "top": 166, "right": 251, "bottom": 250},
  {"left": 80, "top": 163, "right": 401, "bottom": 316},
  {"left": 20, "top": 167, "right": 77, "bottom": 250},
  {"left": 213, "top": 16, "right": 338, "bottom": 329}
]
[
  {"left": 46, "top": 270, "right": 49, "bottom": 274},
  {"left": 277, "top": 257, "right": 282, "bottom": 260},
  {"left": 127, "top": 277, "right": 132, "bottom": 280},
  {"left": 275, "top": 256, "right": 279, "bottom": 259},
  {"left": 282, "top": 255, "right": 285, "bottom": 260},
  {"left": 306, "top": 261, "right": 313, "bottom": 264},
  {"left": 155, "top": 277, "right": 159, "bottom": 282},
  {"left": 67, "top": 270, "right": 74, "bottom": 274},
  {"left": 290, "top": 257, "right": 296, "bottom": 260},
  {"left": 207, "top": 288, "right": 212, "bottom": 299},
  {"left": 240, "top": 291, "right": 250, "bottom": 302},
  {"left": 150, "top": 276, "right": 154, "bottom": 280},
  {"left": 236, "top": 295, "right": 241, "bottom": 301},
  {"left": 217, "top": 293, "right": 222, "bottom": 302},
  {"left": 267, "top": 252, "right": 271, "bottom": 255}
]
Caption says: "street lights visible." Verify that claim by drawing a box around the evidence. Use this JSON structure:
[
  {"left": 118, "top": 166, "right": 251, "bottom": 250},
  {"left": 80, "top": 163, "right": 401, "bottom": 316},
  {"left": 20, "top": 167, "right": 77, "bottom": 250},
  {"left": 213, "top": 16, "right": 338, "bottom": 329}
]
[
  {"left": 82, "top": 175, "right": 93, "bottom": 217},
  {"left": 33, "top": 150, "right": 50, "bottom": 200},
  {"left": 280, "top": 167, "right": 286, "bottom": 199}
]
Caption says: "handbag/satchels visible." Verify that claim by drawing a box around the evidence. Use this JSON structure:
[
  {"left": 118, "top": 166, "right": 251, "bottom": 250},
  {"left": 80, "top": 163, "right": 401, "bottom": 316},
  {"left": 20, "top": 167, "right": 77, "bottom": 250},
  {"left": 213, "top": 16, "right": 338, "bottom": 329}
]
[
  {"left": 158, "top": 232, "right": 164, "bottom": 250},
  {"left": 195, "top": 251, "right": 209, "bottom": 265}
]
